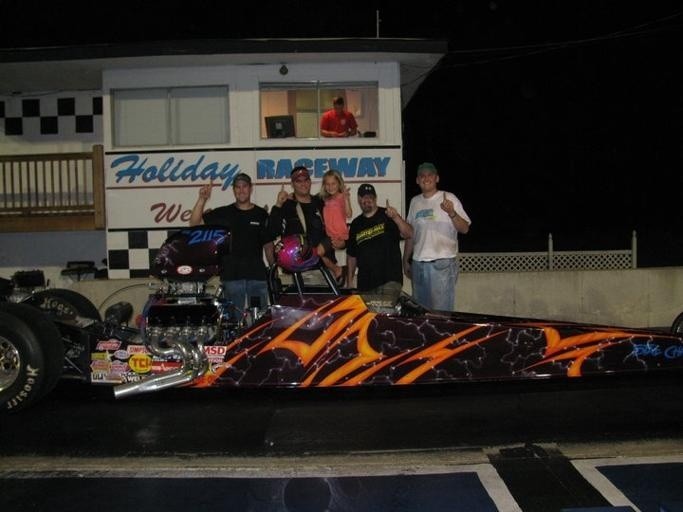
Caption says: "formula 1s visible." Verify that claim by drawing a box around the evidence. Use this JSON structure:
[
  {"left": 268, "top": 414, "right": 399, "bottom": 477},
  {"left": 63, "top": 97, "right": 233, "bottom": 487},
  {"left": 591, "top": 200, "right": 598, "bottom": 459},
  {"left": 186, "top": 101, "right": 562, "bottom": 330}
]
[{"left": 0, "top": 262, "right": 683, "bottom": 454}]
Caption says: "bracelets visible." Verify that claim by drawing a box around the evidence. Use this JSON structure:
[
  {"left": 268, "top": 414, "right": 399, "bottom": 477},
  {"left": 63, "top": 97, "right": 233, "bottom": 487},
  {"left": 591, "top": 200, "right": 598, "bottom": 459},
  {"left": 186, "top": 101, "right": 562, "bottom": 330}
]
[{"left": 448, "top": 212, "right": 457, "bottom": 217}]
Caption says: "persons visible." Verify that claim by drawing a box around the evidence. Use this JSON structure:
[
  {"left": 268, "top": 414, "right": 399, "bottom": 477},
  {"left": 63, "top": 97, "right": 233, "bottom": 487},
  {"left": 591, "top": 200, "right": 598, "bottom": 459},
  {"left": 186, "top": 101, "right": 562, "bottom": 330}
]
[
  {"left": 192, "top": 174, "right": 272, "bottom": 331},
  {"left": 319, "top": 96, "right": 358, "bottom": 137},
  {"left": 346, "top": 184, "right": 411, "bottom": 306},
  {"left": 401, "top": 163, "right": 472, "bottom": 312},
  {"left": 320, "top": 170, "right": 351, "bottom": 284},
  {"left": 267, "top": 167, "right": 335, "bottom": 284}
]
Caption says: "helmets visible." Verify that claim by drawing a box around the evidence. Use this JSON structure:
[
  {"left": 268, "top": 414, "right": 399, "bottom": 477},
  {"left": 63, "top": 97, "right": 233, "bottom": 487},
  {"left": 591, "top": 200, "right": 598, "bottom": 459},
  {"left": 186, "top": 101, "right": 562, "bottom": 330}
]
[{"left": 273, "top": 233, "right": 321, "bottom": 272}]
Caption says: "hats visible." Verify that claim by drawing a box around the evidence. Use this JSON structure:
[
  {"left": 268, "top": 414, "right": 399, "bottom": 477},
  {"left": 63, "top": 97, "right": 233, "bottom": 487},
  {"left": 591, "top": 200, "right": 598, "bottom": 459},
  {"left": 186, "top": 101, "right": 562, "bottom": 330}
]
[
  {"left": 418, "top": 162, "right": 437, "bottom": 175},
  {"left": 234, "top": 174, "right": 251, "bottom": 183},
  {"left": 291, "top": 166, "right": 310, "bottom": 178},
  {"left": 358, "top": 184, "right": 376, "bottom": 197}
]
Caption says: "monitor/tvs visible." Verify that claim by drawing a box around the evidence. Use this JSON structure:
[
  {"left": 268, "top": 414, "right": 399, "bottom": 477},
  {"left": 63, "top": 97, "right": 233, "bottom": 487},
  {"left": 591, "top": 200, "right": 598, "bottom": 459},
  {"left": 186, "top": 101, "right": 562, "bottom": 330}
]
[{"left": 265, "top": 115, "right": 295, "bottom": 140}]
